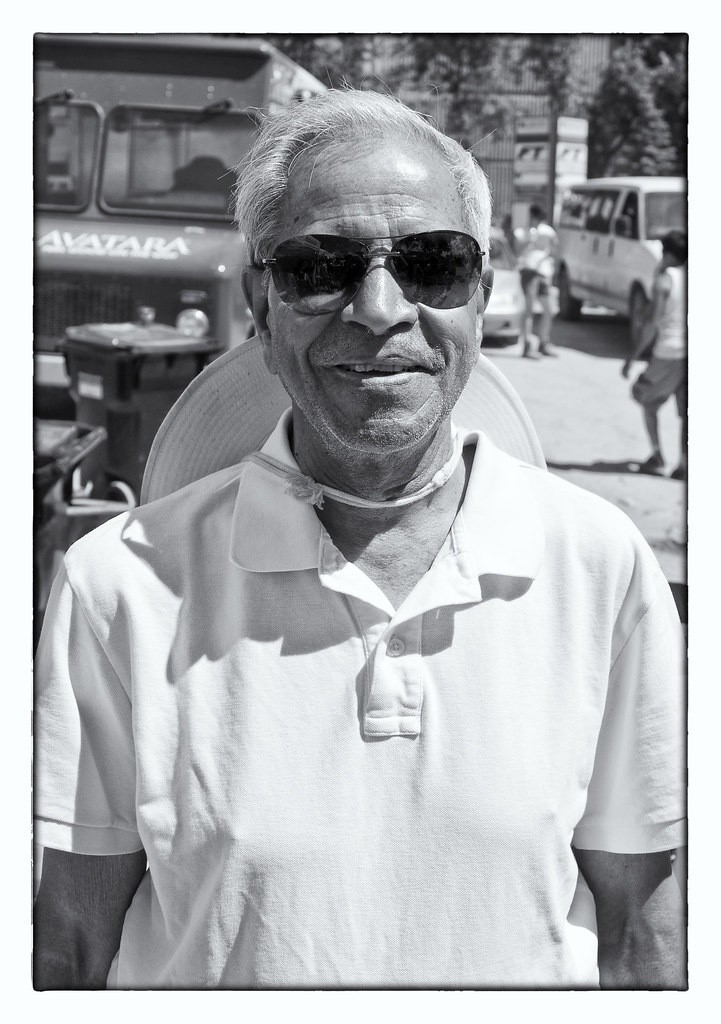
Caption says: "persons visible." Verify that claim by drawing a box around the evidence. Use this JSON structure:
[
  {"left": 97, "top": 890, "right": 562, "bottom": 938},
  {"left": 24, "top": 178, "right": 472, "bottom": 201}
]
[
  {"left": 513, "top": 203, "right": 560, "bottom": 358},
  {"left": 37, "top": 85, "right": 685, "bottom": 988},
  {"left": 621, "top": 230, "right": 685, "bottom": 479}
]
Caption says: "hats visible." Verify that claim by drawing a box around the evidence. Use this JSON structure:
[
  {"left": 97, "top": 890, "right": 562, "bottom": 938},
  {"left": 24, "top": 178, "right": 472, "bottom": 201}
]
[{"left": 139, "top": 332, "right": 548, "bottom": 511}]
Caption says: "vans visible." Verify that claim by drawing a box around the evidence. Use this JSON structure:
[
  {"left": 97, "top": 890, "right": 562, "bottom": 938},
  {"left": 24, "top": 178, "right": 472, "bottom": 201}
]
[{"left": 551, "top": 174, "right": 685, "bottom": 356}]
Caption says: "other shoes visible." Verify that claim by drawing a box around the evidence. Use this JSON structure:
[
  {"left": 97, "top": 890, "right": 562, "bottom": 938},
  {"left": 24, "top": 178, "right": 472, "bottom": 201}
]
[
  {"left": 523, "top": 342, "right": 541, "bottom": 359},
  {"left": 639, "top": 451, "right": 665, "bottom": 473},
  {"left": 670, "top": 467, "right": 688, "bottom": 480},
  {"left": 538, "top": 342, "right": 553, "bottom": 356}
]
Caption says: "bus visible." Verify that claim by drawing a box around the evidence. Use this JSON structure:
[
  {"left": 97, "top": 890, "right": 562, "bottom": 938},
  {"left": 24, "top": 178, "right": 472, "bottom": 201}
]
[{"left": 34, "top": 33, "right": 332, "bottom": 355}]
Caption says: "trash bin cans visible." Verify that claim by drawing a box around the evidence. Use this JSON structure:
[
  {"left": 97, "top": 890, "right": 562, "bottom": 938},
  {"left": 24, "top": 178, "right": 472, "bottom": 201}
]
[{"left": 54, "top": 321, "right": 225, "bottom": 504}]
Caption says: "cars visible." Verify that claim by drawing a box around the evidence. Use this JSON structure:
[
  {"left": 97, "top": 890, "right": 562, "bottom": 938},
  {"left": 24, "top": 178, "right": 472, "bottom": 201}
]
[{"left": 482, "top": 229, "right": 529, "bottom": 342}]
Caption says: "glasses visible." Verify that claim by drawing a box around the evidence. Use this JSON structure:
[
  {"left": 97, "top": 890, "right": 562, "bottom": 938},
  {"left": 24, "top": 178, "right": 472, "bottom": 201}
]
[{"left": 257, "top": 228, "right": 487, "bottom": 317}]
